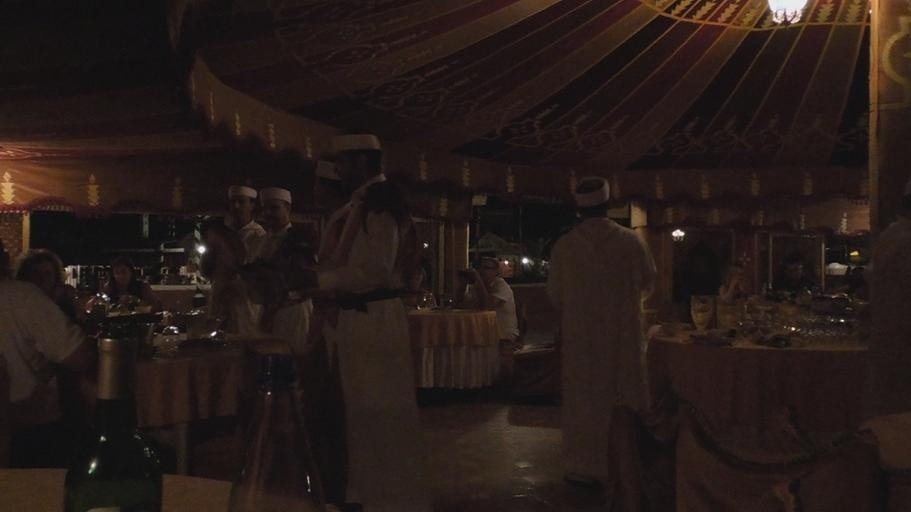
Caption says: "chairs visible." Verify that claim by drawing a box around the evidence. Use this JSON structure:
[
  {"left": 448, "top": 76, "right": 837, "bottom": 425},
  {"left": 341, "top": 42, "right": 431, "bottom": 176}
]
[{"left": 0, "top": 283, "right": 911, "bottom": 511}]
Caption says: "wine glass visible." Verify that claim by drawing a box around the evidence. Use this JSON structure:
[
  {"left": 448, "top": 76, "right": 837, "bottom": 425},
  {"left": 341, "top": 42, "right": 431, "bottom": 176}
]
[{"left": 691, "top": 294, "right": 713, "bottom": 332}]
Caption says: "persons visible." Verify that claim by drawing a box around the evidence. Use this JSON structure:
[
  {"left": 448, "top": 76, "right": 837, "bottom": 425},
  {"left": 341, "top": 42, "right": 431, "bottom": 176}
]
[
  {"left": 318, "top": 134, "right": 418, "bottom": 511},
  {"left": 85, "top": 257, "right": 161, "bottom": 312},
  {"left": 200, "top": 185, "right": 317, "bottom": 353},
  {"left": 473, "top": 258, "right": 519, "bottom": 374},
  {"left": 719, "top": 265, "right": 755, "bottom": 309},
  {"left": 0, "top": 250, "right": 95, "bottom": 428},
  {"left": 546, "top": 176, "right": 656, "bottom": 484}
]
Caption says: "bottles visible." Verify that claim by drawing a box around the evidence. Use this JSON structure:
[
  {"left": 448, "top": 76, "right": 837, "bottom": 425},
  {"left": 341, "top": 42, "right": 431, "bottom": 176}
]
[
  {"left": 226, "top": 354, "right": 328, "bottom": 511},
  {"left": 62, "top": 329, "right": 162, "bottom": 512}
]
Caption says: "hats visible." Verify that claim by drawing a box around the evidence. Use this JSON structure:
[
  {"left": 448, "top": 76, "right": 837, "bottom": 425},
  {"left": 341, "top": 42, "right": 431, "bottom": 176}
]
[
  {"left": 575, "top": 175, "right": 609, "bottom": 206},
  {"left": 328, "top": 132, "right": 379, "bottom": 153},
  {"left": 260, "top": 187, "right": 292, "bottom": 204},
  {"left": 228, "top": 185, "right": 257, "bottom": 198}
]
